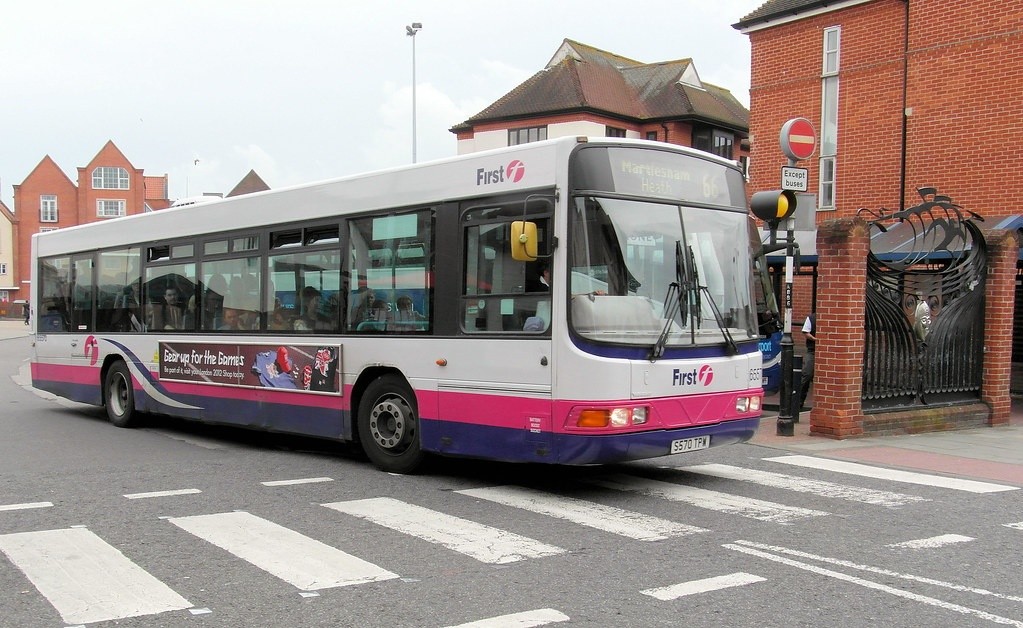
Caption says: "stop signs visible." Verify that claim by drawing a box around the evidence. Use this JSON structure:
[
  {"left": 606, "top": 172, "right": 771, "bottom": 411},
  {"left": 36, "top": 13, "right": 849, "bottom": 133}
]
[
  {"left": 780, "top": 117, "right": 817, "bottom": 161},
  {"left": 781, "top": 119, "right": 819, "bottom": 161}
]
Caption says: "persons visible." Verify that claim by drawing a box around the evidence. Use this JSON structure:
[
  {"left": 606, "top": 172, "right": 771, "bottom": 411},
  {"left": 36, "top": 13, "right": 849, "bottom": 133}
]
[
  {"left": 21, "top": 300, "right": 30, "bottom": 325},
  {"left": 397, "top": 296, "right": 426, "bottom": 330},
  {"left": 359, "top": 287, "right": 393, "bottom": 330},
  {"left": 797, "top": 312, "right": 816, "bottom": 411},
  {"left": 529, "top": 253, "right": 606, "bottom": 295},
  {"left": 126, "top": 272, "right": 289, "bottom": 330},
  {"left": 293, "top": 286, "right": 339, "bottom": 331}
]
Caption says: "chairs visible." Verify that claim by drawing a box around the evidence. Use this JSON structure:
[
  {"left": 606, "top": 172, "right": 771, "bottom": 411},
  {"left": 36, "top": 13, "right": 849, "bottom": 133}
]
[{"left": 212, "top": 311, "right": 224, "bottom": 329}]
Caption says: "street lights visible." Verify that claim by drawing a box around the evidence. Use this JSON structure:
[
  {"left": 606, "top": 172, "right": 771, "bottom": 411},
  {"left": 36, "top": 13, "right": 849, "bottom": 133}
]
[{"left": 405, "top": 22, "right": 422, "bottom": 163}]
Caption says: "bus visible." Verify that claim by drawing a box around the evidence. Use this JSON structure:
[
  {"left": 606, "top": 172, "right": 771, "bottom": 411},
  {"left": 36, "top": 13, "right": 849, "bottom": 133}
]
[{"left": 29, "top": 128, "right": 802, "bottom": 474}]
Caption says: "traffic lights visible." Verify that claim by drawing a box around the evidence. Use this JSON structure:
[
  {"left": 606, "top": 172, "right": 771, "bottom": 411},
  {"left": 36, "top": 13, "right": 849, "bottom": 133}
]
[{"left": 750, "top": 188, "right": 797, "bottom": 222}]
